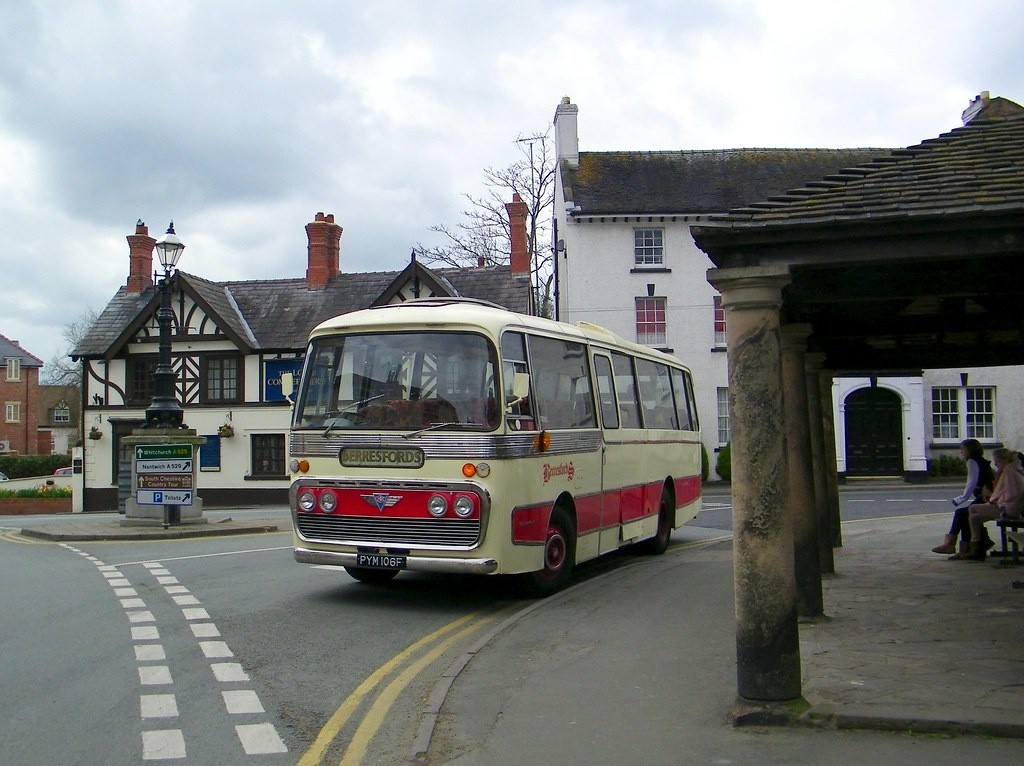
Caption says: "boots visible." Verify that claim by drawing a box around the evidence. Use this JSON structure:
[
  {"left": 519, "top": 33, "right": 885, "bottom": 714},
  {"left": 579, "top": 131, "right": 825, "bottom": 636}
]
[
  {"left": 949, "top": 541, "right": 970, "bottom": 560},
  {"left": 982, "top": 527, "right": 995, "bottom": 550},
  {"left": 932, "top": 533, "right": 958, "bottom": 553},
  {"left": 960, "top": 542, "right": 985, "bottom": 562}
]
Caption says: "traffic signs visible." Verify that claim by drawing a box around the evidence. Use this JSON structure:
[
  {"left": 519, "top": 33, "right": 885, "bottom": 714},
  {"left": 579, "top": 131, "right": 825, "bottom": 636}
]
[{"left": 134, "top": 442, "right": 195, "bottom": 506}]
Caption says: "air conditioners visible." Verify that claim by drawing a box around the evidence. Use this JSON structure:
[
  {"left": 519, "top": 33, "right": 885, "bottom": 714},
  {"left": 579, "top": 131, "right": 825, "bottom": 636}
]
[{"left": 0, "top": 440, "right": 10, "bottom": 452}]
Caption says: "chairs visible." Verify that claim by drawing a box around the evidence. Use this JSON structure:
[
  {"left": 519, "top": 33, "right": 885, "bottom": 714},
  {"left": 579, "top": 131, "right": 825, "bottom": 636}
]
[{"left": 356, "top": 396, "right": 689, "bottom": 430}]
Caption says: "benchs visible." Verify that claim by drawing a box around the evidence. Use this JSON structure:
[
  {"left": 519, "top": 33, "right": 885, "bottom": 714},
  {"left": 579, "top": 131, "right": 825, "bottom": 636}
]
[
  {"left": 991, "top": 518, "right": 1024, "bottom": 564},
  {"left": 1005, "top": 531, "right": 1024, "bottom": 588}
]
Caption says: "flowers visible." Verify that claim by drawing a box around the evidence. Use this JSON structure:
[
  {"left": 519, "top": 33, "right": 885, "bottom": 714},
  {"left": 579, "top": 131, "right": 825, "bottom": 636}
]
[
  {"left": 89, "top": 426, "right": 103, "bottom": 440},
  {"left": 217, "top": 424, "right": 235, "bottom": 438}
]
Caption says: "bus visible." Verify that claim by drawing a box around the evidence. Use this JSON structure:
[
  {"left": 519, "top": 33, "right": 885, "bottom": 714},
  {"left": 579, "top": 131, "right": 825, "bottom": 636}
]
[{"left": 284, "top": 294, "right": 705, "bottom": 597}]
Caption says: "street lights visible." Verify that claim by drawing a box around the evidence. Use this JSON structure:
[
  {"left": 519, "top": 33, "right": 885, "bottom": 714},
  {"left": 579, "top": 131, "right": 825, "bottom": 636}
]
[{"left": 140, "top": 218, "right": 191, "bottom": 429}]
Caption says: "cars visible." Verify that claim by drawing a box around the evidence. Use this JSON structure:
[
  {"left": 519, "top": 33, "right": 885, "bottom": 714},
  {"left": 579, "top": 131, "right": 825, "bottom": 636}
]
[
  {"left": 0, "top": 472, "right": 9, "bottom": 481},
  {"left": 55, "top": 467, "right": 72, "bottom": 474}
]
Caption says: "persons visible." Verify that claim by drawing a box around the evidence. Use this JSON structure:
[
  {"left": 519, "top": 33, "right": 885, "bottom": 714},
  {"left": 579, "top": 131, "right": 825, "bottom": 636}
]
[
  {"left": 961, "top": 448, "right": 1024, "bottom": 561},
  {"left": 931, "top": 438, "right": 995, "bottom": 560}
]
